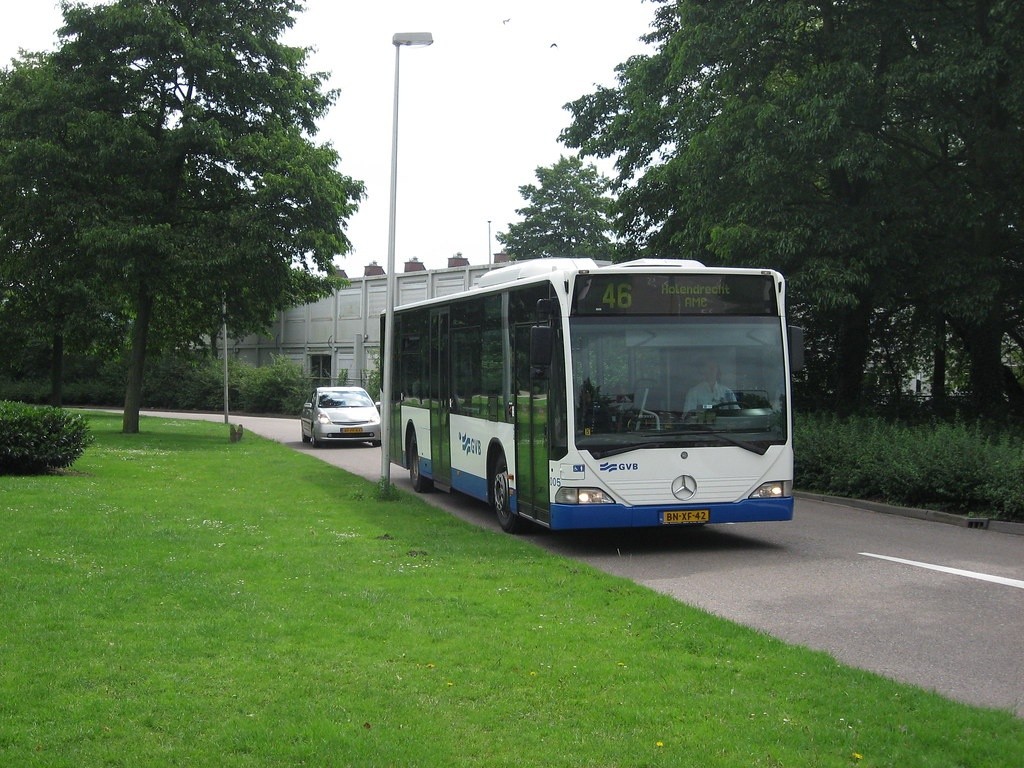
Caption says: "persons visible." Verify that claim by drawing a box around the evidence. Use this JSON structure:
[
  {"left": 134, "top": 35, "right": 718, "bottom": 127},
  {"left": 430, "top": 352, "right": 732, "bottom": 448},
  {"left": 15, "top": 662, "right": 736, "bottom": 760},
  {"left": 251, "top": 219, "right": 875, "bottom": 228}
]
[
  {"left": 412, "top": 369, "right": 430, "bottom": 397},
  {"left": 681, "top": 358, "right": 741, "bottom": 423}
]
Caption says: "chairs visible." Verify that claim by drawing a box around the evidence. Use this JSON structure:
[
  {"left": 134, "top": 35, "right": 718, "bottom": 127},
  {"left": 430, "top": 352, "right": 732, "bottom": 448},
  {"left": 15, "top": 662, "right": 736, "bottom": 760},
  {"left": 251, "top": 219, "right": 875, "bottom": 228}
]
[{"left": 633, "top": 378, "right": 658, "bottom": 403}]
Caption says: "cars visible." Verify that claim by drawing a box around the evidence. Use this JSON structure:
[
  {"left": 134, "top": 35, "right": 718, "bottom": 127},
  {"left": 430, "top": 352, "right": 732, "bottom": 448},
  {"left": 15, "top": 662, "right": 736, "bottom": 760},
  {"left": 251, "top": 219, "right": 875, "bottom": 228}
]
[{"left": 301, "top": 387, "right": 381, "bottom": 448}]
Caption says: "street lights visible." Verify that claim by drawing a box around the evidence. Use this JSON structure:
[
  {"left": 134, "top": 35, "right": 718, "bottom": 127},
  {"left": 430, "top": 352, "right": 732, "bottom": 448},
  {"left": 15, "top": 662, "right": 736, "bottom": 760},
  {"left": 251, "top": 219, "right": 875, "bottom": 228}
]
[
  {"left": 487, "top": 220, "right": 492, "bottom": 271},
  {"left": 378, "top": 31, "right": 436, "bottom": 500}
]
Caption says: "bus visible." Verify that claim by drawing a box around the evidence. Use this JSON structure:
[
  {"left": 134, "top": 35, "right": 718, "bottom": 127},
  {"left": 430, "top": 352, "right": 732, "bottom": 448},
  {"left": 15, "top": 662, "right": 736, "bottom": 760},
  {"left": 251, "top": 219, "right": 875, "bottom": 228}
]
[{"left": 374, "top": 254, "right": 805, "bottom": 536}]
